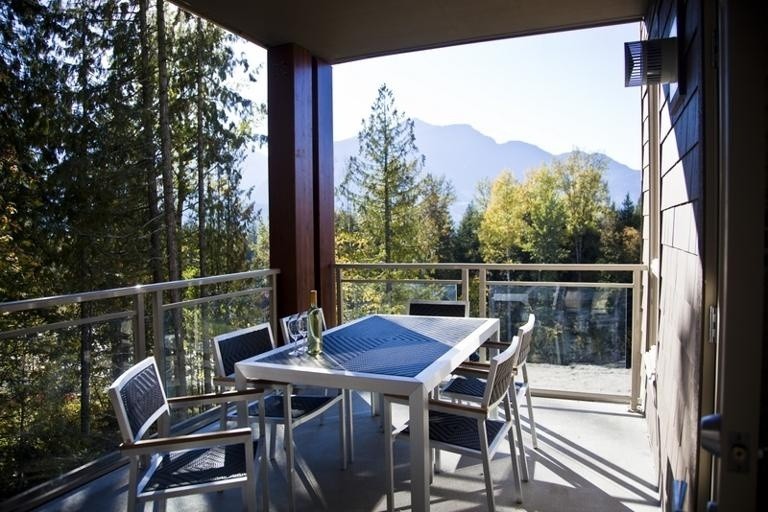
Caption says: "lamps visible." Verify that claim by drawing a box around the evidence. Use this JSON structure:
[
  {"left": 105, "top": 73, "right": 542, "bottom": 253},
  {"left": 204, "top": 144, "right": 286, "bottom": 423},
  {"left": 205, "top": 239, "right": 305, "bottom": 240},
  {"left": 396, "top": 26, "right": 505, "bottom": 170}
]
[{"left": 624, "top": 36, "right": 679, "bottom": 88}]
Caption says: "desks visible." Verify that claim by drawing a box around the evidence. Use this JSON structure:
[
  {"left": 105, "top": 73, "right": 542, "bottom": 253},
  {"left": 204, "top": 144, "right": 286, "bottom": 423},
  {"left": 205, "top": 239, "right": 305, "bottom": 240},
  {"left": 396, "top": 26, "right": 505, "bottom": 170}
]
[{"left": 234, "top": 315, "right": 502, "bottom": 511}]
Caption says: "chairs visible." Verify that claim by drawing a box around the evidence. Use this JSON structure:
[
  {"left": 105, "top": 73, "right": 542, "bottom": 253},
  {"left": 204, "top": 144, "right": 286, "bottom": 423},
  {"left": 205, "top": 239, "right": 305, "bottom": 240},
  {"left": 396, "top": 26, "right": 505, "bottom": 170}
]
[
  {"left": 280, "top": 308, "right": 378, "bottom": 462},
  {"left": 407, "top": 300, "right": 469, "bottom": 317},
  {"left": 383, "top": 336, "right": 524, "bottom": 511},
  {"left": 435, "top": 313, "right": 538, "bottom": 481},
  {"left": 212, "top": 321, "right": 347, "bottom": 471},
  {"left": 105, "top": 357, "right": 270, "bottom": 512}
]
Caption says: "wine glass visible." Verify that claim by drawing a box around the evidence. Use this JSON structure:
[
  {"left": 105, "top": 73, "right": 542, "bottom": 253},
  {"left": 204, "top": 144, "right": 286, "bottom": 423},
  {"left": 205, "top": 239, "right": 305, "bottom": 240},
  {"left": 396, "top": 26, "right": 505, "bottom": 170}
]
[
  {"left": 287, "top": 319, "right": 304, "bottom": 357},
  {"left": 296, "top": 312, "right": 309, "bottom": 352}
]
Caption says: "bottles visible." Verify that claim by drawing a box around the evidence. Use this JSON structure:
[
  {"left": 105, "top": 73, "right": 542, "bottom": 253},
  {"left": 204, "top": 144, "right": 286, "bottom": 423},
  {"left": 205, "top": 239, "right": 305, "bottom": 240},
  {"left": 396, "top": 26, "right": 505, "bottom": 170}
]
[{"left": 308, "top": 289, "right": 323, "bottom": 355}]
